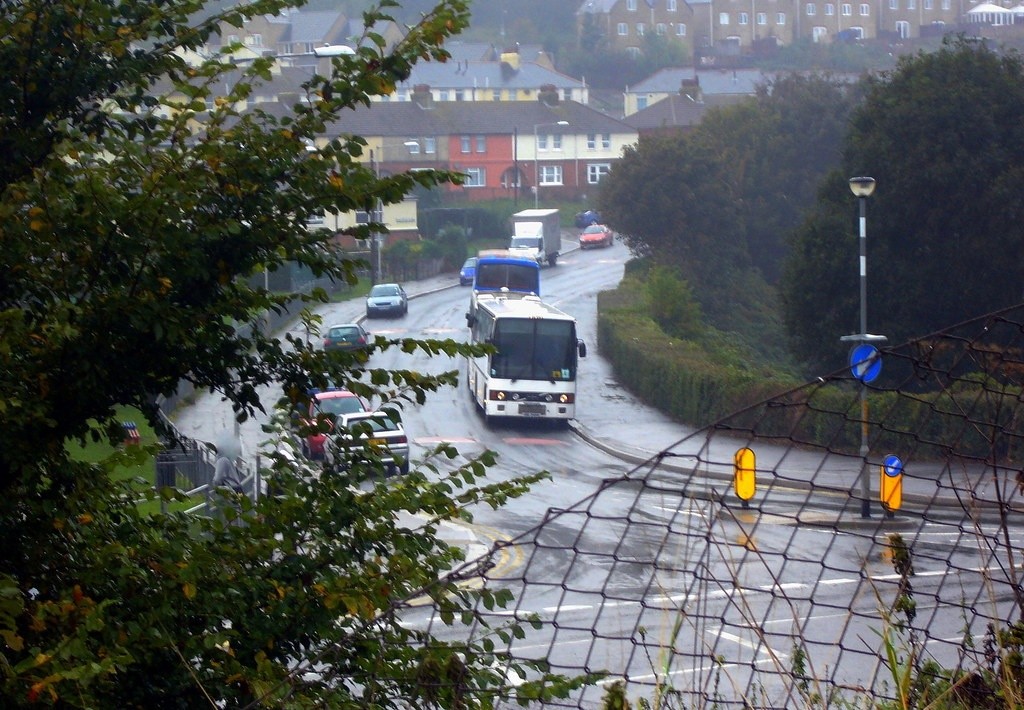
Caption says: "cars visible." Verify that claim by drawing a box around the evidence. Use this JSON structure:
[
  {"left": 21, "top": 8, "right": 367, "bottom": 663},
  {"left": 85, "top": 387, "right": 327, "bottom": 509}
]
[
  {"left": 459, "top": 258, "right": 476, "bottom": 287},
  {"left": 578, "top": 224, "right": 614, "bottom": 249},
  {"left": 322, "top": 323, "right": 372, "bottom": 361},
  {"left": 298, "top": 390, "right": 373, "bottom": 462},
  {"left": 576, "top": 209, "right": 604, "bottom": 228},
  {"left": 324, "top": 411, "right": 410, "bottom": 482},
  {"left": 287, "top": 370, "right": 348, "bottom": 428},
  {"left": 363, "top": 283, "right": 409, "bottom": 318}
]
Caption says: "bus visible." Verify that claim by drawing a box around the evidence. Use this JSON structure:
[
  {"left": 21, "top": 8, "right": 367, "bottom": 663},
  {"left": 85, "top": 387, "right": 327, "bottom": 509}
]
[{"left": 468, "top": 249, "right": 587, "bottom": 425}]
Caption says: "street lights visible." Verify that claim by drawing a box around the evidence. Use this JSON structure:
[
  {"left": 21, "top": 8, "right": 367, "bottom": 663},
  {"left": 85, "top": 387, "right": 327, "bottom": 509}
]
[{"left": 849, "top": 177, "right": 877, "bottom": 521}]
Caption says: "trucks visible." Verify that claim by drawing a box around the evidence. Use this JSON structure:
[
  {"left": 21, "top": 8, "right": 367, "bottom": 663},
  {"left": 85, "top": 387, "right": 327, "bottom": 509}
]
[{"left": 509, "top": 208, "right": 563, "bottom": 267}]
